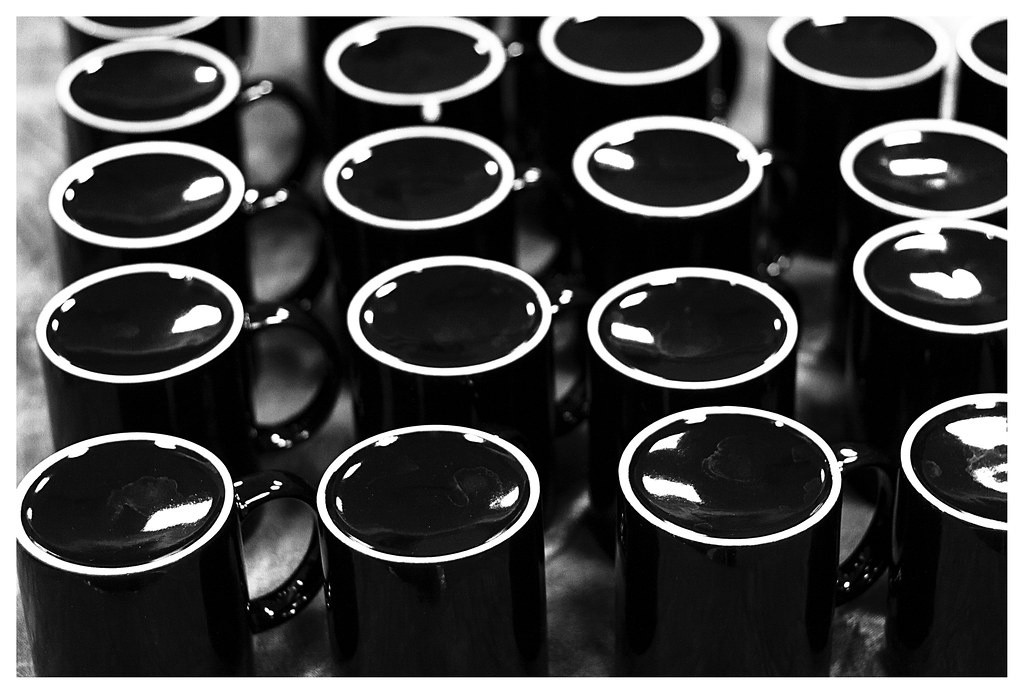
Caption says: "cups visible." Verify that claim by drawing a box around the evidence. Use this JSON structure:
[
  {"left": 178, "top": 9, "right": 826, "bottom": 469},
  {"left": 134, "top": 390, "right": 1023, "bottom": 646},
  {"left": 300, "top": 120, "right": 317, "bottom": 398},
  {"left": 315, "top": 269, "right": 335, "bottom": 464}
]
[
  {"left": 878, "top": 393, "right": 1007, "bottom": 677},
  {"left": 323, "top": 18, "right": 529, "bottom": 167},
  {"left": 585, "top": 266, "right": 804, "bottom": 561},
  {"left": 48, "top": 141, "right": 335, "bottom": 312},
  {"left": 612, "top": 408, "right": 897, "bottom": 677},
  {"left": 34, "top": 262, "right": 343, "bottom": 459},
  {"left": 58, "top": 16, "right": 254, "bottom": 65},
  {"left": 838, "top": 118, "right": 1007, "bottom": 306},
  {"left": 346, "top": 254, "right": 590, "bottom": 516},
  {"left": 570, "top": 115, "right": 806, "bottom": 301},
  {"left": 56, "top": 39, "right": 320, "bottom": 219},
  {"left": 955, "top": 18, "right": 1007, "bottom": 138},
  {"left": 770, "top": 16, "right": 943, "bottom": 265},
  {"left": 16, "top": 432, "right": 324, "bottom": 677},
  {"left": 300, "top": 16, "right": 505, "bottom": 116},
  {"left": 537, "top": 19, "right": 740, "bottom": 180},
  {"left": 844, "top": 219, "right": 1007, "bottom": 446},
  {"left": 320, "top": 125, "right": 573, "bottom": 351},
  {"left": 315, "top": 424, "right": 549, "bottom": 676}
]
